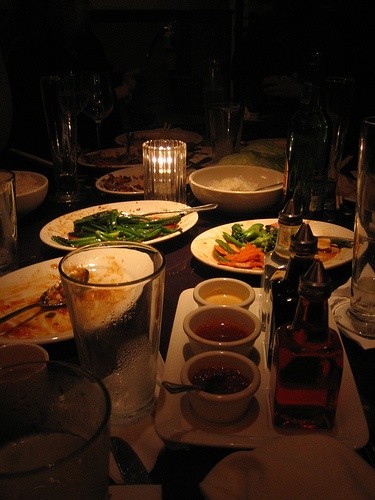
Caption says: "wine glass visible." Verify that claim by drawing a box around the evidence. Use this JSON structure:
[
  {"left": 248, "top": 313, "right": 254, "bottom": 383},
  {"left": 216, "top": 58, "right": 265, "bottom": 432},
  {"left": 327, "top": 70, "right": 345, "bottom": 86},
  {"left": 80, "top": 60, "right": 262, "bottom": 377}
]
[{"left": 74, "top": 73, "right": 117, "bottom": 159}]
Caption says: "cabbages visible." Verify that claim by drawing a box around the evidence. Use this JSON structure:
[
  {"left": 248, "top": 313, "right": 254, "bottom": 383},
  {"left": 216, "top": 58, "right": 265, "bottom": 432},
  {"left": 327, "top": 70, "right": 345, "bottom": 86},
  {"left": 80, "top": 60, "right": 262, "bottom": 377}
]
[{"left": 213, "top": 139, "right": 287, "bottom": 169}]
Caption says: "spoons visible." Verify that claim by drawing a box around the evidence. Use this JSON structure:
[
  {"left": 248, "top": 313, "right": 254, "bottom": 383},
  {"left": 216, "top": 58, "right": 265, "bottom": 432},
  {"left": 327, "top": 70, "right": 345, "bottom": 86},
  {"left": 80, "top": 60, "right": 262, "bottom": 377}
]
[{"left": 0, "top": 268, "right": 89, "bottom": 324}]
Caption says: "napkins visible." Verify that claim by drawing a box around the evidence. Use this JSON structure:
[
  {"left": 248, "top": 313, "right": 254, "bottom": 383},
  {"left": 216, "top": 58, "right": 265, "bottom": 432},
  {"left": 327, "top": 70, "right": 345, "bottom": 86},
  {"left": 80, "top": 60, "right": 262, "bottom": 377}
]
[
  {"left": 199, "top": 434, "right": 375, "bottom": 500},
  {"left": 328, "top": 263, "right": 375, "bottom": 350}
]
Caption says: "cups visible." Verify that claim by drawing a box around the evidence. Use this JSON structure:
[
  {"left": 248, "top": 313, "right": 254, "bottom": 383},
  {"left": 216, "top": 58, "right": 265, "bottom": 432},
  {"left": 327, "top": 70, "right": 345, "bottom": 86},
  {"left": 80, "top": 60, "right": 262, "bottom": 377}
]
[
  {"left": 39, "top": 73, "right": 77, "bottom": 203},
  {"left": 323, "top": 179, "right": 338, "bottom": 222},
  {"left": 0, "top": 172, "right": 18, "bottom": 269},
  {"left": 58, "top": 240, "right": 167, "bottom": 427},
  {"left": 0, "top": 363, "right": 113, "bottom": 500},
  {"left": 208, "top": 103, "right": 244, "bottom": 161},
  {"left": 334, "top": 116, "right": 374, "bottom": 340},
  {"left": 1, "top": 342, "right": 50, "bottom": 425},
  {"left": 322, "top": 75, "right": 354, "bottom": 223}
]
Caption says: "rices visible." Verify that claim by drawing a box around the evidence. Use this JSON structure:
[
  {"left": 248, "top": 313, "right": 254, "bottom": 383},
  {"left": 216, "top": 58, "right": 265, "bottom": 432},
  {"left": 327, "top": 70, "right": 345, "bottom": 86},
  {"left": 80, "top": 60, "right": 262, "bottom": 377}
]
[
  {"left": 12, "top": 173, "right": 41, "bottom": 195},
  {"left": 206, "top": 175, "right": 266, "bottom": 193}
]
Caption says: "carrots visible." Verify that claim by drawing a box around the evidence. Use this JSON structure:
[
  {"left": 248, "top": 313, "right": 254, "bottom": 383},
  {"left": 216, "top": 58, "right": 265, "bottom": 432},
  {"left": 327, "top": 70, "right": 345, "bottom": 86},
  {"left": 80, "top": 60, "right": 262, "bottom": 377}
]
[{"left": 213, "top": 243, "right": 265, "bottom": 269}]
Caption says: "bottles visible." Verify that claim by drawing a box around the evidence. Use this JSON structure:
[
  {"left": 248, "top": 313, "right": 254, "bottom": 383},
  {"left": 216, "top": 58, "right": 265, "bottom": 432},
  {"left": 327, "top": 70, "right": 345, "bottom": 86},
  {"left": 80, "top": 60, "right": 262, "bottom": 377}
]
[
  {"left": 267, "top": 281, "right": 346, "bottom": 432},
  {"left": 259, "top": 201, "right": 303, "bottom": 328},
  {"left": 282, "top": 59, "right": 334, "bottom": 219},
  {"left": 268, "top": 224, "right": 332, "bottom": 362}
]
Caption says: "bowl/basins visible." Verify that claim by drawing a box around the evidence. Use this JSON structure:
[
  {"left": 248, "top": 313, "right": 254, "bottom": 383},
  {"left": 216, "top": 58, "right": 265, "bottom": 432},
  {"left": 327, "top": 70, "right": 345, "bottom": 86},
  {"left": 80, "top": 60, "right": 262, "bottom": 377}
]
[
  {"left": 0, "top": 170, "right": 49, "bottom": 212},
  {"left": 188, "top": 165, "right": 287, "bottom": 210},
  {"left": 194, "top": 277, "right": 257, "bottom": 309},
  {"left": 185, "top": 308, "right": 263, "bottom": 356},
  {"left": 182, "top": 352, "right": 262, "bottom": 422}
]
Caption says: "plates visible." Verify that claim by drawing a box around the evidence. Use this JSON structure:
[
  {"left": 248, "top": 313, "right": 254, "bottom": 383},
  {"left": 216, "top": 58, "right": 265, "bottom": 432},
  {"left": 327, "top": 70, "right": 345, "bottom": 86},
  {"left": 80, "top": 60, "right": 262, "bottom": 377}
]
[
  {"left": 93, "top": 163, "right": 198, "bottom": 196},
  {"left": 190, "top": 217, "right": 367, "bottom": 277},
  {"left": 79, "top": 141, "right": 212, "bottom": 167},
  {"left": 115, "top": 127, "right": 205, "bottom": 148},
  {"left": 38, "top": 199, "right": 199, "bottom": 250},
  {"left": 1, "top": 248, "right": 155, "bottom": 344},
  {"left": 244, "top": 137, "right": 288, "bottom": 169}
]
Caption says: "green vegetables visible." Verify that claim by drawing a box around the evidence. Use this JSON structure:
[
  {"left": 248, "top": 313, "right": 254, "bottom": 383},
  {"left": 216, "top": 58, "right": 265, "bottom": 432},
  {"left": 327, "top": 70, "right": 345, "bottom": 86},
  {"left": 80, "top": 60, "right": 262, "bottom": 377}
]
[
  {"left": 51, "top": 209, "right": 182, "bottom": 248},
  {"left": 215, "top": 223, "right": 277, "bottom": 261},
  {"left": 329, "top": 238, "right": 355, "bottom": 249}
]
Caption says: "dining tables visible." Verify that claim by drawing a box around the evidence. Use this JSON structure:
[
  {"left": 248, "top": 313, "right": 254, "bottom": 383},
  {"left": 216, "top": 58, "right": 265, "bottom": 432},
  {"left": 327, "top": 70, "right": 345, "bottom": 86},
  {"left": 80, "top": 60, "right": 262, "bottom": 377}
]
[{"left": 0, "top": 125, "right": 375, "bottom": 500}]
